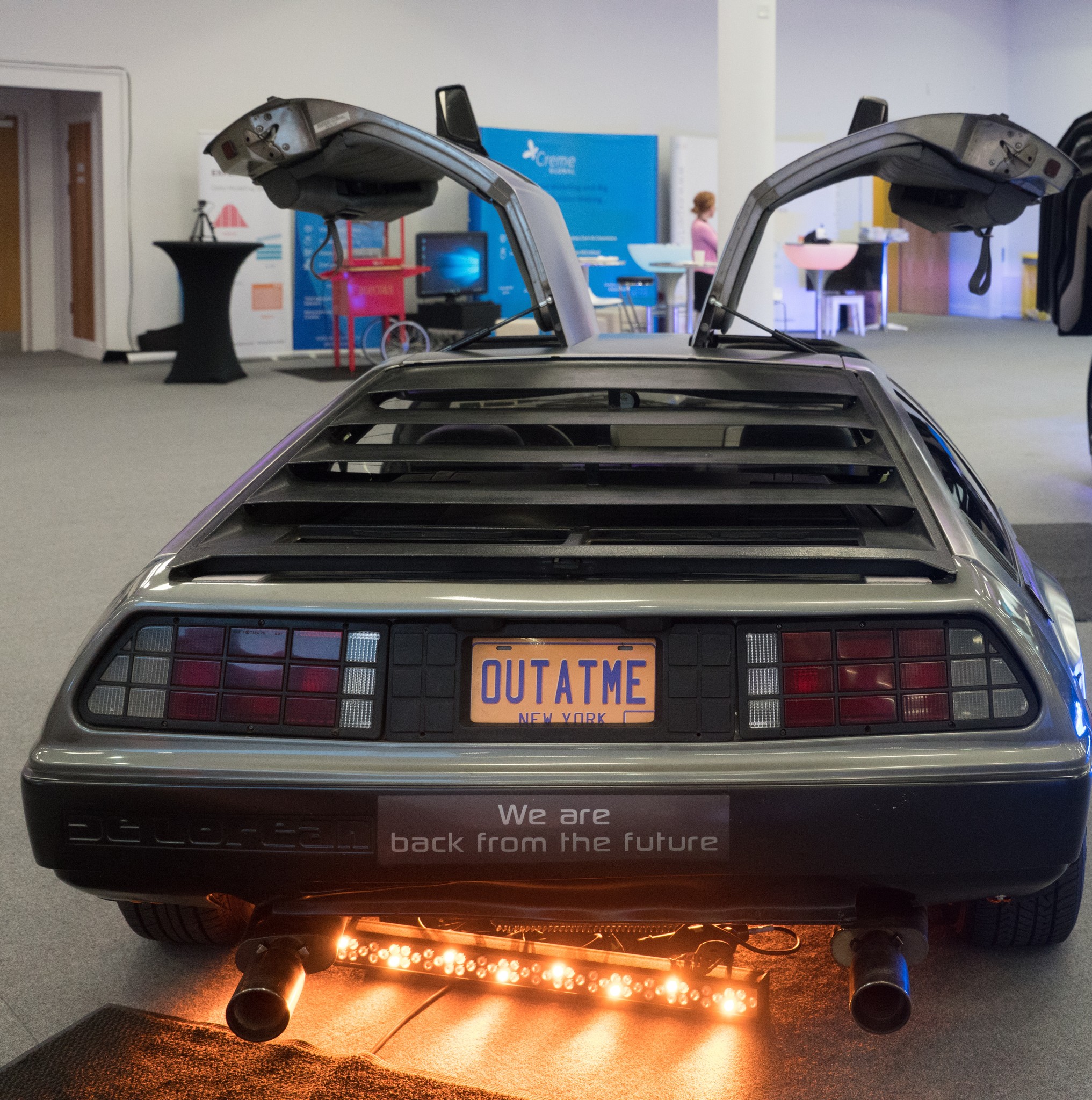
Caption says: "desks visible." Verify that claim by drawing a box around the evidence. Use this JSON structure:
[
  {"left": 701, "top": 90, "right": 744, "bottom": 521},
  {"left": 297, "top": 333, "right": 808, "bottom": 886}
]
[
  {"left": 152, "top": 241, "right": 264, "bottom": 384},
  {"left": 578, "top": 257, "right": 625, "bottom": 286},
  {"left": 651, "top": 263, "right": 719, "bottom": 333},
  {"left": 783, "top": 227, "right": 910, "bottom": 341},
  {"left": 320, "top": 266, "right": 431, "bottom": 373}
]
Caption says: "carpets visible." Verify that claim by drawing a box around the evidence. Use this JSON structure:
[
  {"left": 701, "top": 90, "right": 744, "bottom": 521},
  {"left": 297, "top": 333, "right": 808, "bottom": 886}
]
[{"left": 0, "top": 1002, "right": 545, "bottom": 1100}]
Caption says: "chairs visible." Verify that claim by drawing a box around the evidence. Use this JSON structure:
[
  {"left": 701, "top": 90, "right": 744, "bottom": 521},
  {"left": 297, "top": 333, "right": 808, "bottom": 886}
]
[
  {"left": 588, "top": 286, "right": 623, "bottom": 333},
  {"left": 409, "top": 425, "right": 531, "bottom": 472},
  {"left": 739, "top": 406, "right": 856, "bottom": 475}
]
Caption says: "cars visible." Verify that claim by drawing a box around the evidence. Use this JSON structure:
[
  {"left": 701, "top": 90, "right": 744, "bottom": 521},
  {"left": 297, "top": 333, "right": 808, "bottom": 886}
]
[{"left": 21, "top": 86, "right": 1092, "bottom": 1043}]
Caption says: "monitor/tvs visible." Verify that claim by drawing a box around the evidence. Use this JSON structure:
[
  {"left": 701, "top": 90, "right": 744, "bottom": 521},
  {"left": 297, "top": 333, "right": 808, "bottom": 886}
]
[
  {"left": 806, "top": 240, "right": 885, "bottom": 292},
  {"left": 418, "top": 231, "right": 490, "bottom": 298}
]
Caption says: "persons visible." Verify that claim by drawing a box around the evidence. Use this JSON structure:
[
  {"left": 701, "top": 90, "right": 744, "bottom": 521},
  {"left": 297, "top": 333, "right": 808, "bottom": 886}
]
[{"left": 691, "top": 192, "right": 719, "bottom": 330}]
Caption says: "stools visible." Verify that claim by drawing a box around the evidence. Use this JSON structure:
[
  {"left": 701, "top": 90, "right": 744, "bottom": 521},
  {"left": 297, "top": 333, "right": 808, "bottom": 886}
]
[
  {"left": 617, "top": 277, "right": 655, "bottom": 334},
  {"left": 822, "top": 294, "right": 866, "bottom": 337}
]
